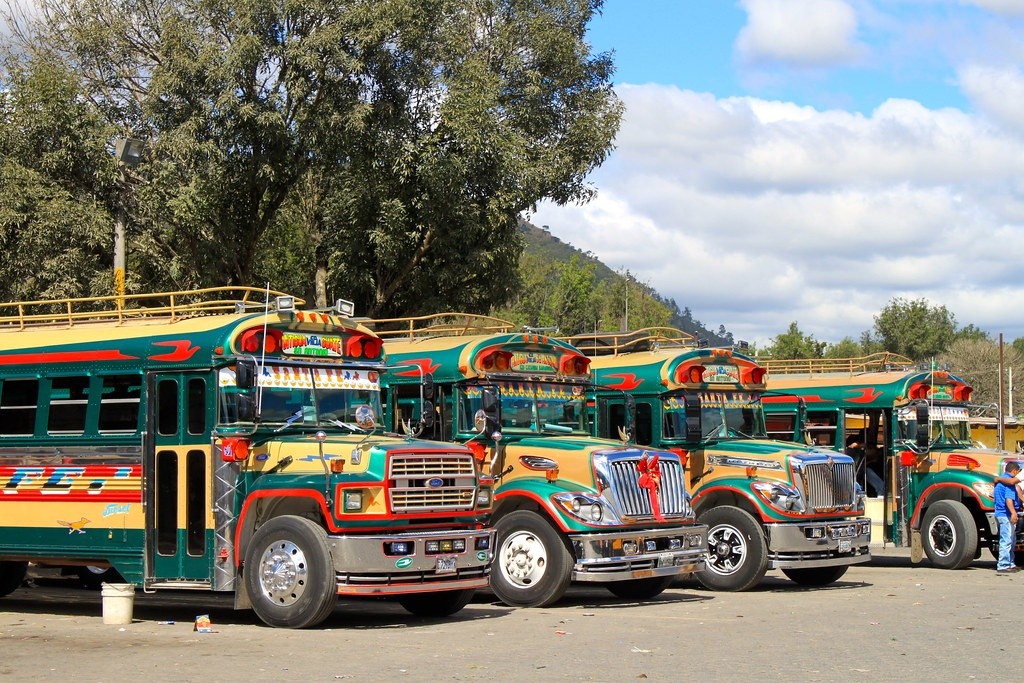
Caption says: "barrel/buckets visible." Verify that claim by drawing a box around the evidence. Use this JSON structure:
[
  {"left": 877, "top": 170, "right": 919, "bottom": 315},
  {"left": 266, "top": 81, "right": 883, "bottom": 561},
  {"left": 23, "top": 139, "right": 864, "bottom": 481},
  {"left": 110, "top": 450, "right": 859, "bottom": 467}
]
[{"left": 99, "top": 581, "right": 135, "bottom": 625}]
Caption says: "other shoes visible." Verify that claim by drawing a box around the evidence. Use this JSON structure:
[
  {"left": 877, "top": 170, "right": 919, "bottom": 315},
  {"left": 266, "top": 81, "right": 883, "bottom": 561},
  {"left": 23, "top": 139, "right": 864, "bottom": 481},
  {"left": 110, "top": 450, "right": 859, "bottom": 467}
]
[{"left": 996, "top": 565, "right": 1021, "bottom": 575}]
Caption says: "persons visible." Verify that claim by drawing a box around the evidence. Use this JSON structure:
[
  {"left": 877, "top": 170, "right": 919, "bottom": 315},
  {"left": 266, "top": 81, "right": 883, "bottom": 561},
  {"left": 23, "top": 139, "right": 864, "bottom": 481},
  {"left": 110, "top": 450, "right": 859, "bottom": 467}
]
[
  {"left": 994, "top": 462, "right": 1024, "bottom": 572},
  {"left": 813, "top": 424, "right": 885, "bottom": 497}
]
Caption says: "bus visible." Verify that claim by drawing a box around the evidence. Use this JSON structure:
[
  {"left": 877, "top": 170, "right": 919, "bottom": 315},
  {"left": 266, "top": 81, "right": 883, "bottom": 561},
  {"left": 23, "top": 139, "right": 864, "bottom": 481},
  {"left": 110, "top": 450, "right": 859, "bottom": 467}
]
[
  {"left": 0, "top": 289, "right": 501, "bottom": 629},
  {"left": 744, "top": 353, "right": 1024, "bottom": 569},
  {"left": 362, "top": 318, "right": 712, "bottom": 612},
  {"left": 549, "top": 328, "right": 865, "bottom": 591}
]
